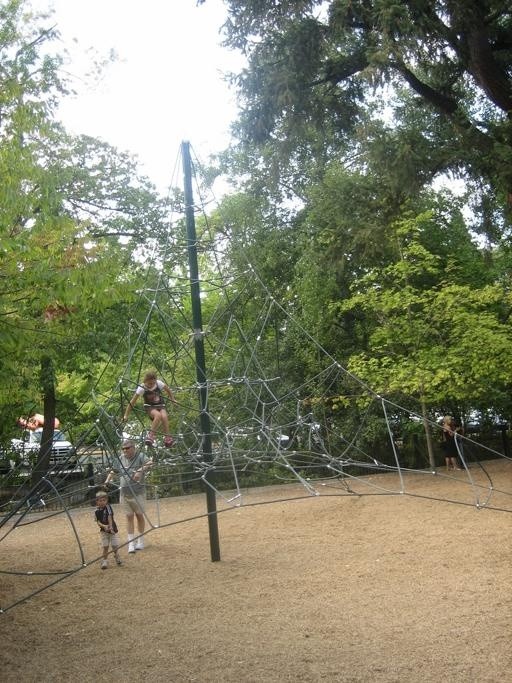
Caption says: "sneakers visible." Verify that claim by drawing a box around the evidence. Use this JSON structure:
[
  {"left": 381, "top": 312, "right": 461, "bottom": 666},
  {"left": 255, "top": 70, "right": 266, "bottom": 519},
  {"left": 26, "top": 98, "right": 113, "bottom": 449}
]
[
  {"left": 116, "top": 557, "right": 121, "bottom": 564},
  {"left": 145, "top": 439, "right": 173, "bottom": 447},
  {"left": 127, "top": 544, "right": 135, "bottom": 552},
  {"left": 135, "top": 540, "right": 144, "bottom": 550},
  {"left": 100, "top": 560, "right": 108, "bottom": 568}
]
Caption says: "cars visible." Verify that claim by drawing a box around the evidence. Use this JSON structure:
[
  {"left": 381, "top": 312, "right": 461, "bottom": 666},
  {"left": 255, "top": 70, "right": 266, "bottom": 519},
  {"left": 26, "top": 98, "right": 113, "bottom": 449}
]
[{"left": 225, "top": 424, "right": 290, "bottom": 445}]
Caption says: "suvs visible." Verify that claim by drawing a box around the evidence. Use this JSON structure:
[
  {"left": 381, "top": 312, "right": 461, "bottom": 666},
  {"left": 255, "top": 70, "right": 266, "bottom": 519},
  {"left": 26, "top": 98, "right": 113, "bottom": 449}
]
[
  {"left": 11, "top": 427, "right": 76, "bottom": 469},
  {"left": 119, "top": 421, "right": 163, "bottom": 453}
]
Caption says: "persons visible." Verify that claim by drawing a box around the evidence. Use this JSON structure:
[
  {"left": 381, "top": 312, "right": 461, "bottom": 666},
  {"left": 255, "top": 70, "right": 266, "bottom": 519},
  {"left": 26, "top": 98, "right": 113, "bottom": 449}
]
[
  {"left": 437, "top": 414, "right": 463, "bottom": 471},
  {"left": 93, "top": 489, "right": 125, "bottom": 571},
  {"left": 103, "top": 436, "right": 153, "bottom": 554},
  {"left": 120, "top": 370, "right": 178, "bottom": 448}
]
